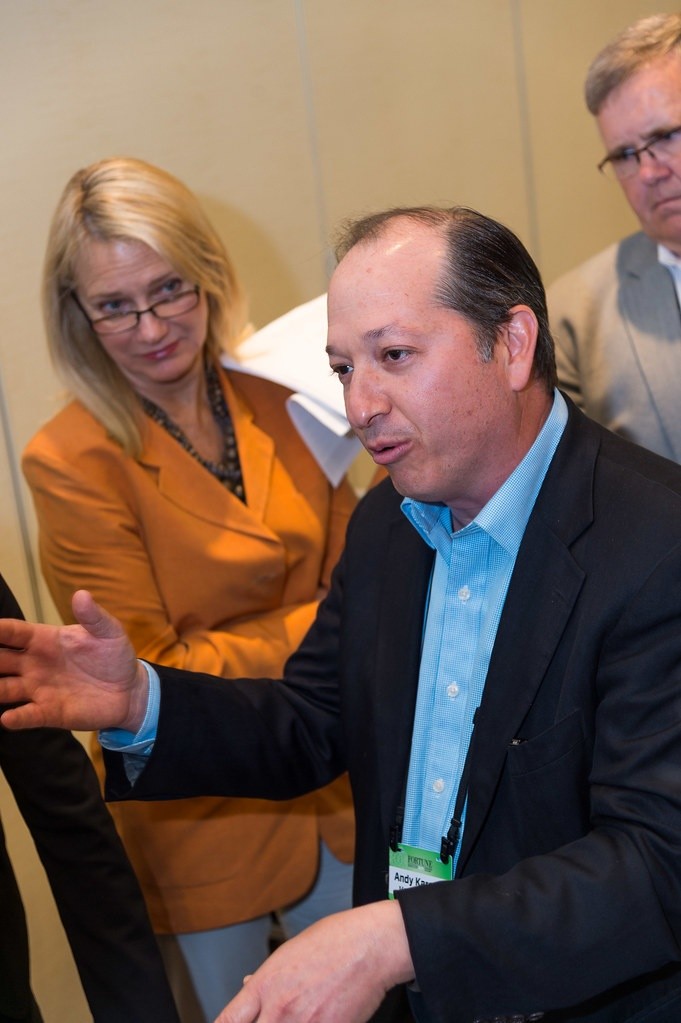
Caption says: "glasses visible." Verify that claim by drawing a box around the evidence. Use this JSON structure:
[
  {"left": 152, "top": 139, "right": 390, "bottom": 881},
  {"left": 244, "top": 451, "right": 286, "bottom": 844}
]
[
  {"left": 69, "top": 263, "right": 200, "bottom": 337},
  {"left": 598, "top": 125, "right": 681, "bottom": 182}
]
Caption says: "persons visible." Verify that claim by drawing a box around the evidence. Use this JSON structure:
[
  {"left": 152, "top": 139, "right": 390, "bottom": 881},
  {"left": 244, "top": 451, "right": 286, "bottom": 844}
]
[{"left": 0, "top": 12, "right": 681, "bottom": 1023}]
